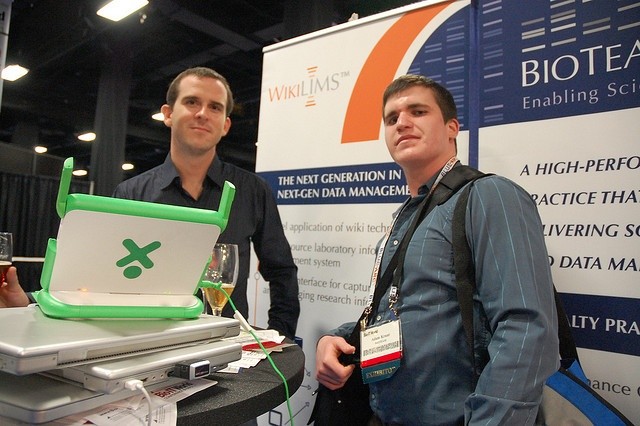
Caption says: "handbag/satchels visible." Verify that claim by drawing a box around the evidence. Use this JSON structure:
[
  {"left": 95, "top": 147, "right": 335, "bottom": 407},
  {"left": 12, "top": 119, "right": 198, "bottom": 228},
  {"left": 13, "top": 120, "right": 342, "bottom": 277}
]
[
  {"left": 306, "top": 164, "right": 484, "bottom": 426},
  {"left": 452, "top": 173, "right": 635, "bottom": 426}
]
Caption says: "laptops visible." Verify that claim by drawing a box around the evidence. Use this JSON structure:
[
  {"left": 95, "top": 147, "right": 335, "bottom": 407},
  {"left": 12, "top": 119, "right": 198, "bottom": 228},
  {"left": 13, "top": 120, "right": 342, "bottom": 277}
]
[
  {"left": 0, "top": 362, "right": 234, "bottom": 424},
  {"left": 2, "top": 313, "right": 242, "bottom": 378},
  {"left": 78, "top": 342, "right": 262, "bottom": 396}
]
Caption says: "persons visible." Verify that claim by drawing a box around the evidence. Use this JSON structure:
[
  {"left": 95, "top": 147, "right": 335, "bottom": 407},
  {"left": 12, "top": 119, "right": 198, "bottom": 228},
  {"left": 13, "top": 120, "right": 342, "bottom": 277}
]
[
  {"left": 314, "top": 74, "right": 561, "bottom": 426},
  {"left": 0, "top": 66, "right": 300, "bottom": 343}
]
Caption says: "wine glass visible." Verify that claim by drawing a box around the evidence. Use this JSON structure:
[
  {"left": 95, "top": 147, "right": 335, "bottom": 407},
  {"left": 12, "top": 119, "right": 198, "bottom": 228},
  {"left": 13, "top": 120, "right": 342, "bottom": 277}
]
[
  {"left": 1, "top": 232, "right": 12, "bottom": 281},
  {"left": 201, "top": 243, "right": 240, "bottom": 315}
]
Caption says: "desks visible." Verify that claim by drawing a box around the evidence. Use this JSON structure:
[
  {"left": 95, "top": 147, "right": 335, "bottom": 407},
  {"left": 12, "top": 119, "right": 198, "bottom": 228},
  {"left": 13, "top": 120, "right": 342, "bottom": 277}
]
[{"left": 1, "top": 325, "right": 305, "bottom": 426}]
